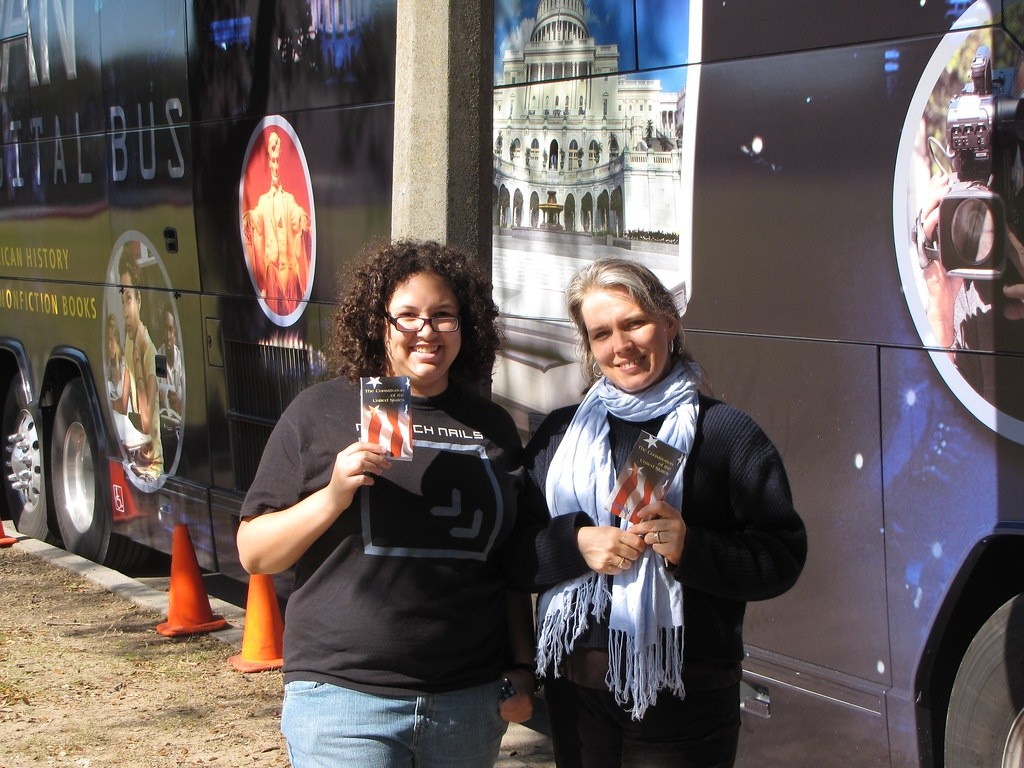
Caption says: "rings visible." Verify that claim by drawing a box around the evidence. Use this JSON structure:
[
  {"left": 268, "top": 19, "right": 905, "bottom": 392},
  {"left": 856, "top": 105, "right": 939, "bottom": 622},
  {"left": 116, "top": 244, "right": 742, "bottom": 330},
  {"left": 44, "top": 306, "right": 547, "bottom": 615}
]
[
  {"left": 654, "top": 531, "right": 660, "bottom": 543},
  {"left": 617, "top": 558, "right": 625, "bottom": 568}
]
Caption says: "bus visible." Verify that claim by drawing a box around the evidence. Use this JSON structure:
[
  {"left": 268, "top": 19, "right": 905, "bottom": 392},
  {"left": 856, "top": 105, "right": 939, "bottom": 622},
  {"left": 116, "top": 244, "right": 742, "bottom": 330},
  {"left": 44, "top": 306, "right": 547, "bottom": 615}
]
[{"left": 0, "top": 0, "right": 1024, "bottom": 768}]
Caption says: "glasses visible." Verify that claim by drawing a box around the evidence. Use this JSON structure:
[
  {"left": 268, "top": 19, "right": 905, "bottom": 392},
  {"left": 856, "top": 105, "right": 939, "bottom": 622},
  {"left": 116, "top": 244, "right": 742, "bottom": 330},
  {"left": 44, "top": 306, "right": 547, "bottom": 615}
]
[{"left": 384, "top": 311, "right": 463, "bottom": 332}]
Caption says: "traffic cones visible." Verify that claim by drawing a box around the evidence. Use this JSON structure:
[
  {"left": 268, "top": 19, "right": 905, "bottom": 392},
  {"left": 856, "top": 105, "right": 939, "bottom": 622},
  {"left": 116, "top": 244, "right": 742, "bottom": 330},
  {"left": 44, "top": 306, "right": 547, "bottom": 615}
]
[
  {"left": 227, "top": 571, "right": 289, "bottom": 674},
  {"left": 155, "top": 520, "right": 227, "bottom": 637}
]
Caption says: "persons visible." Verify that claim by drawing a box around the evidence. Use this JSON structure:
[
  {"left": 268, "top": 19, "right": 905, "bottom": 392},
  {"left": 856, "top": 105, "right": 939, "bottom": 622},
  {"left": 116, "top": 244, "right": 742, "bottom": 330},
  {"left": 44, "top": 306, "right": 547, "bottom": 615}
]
[
  {"left": 234, "top": 239, "right": 534, "bottom": 768},
  {"left": 243, "top": 131, "right": 311, "bottom": 314},
  {"left": 910, "top": 63, "right": 1024, "bottom": 420},
  {"left": 102, "top": 250, "right": 182, "bottom": 485},
  {"left": 521, "top": 258, "right": 807, "bottom": 768}
]
